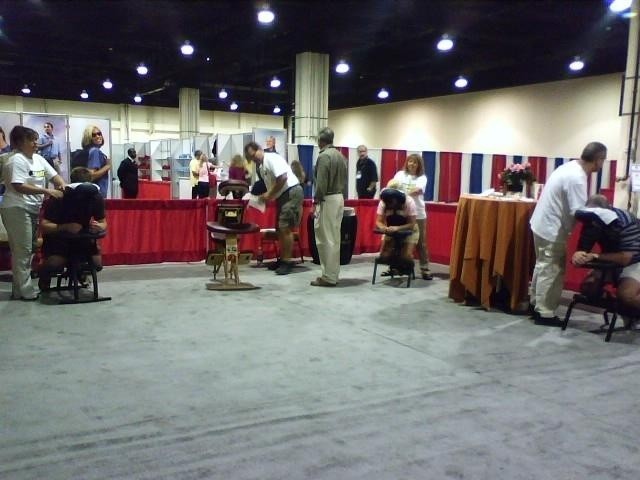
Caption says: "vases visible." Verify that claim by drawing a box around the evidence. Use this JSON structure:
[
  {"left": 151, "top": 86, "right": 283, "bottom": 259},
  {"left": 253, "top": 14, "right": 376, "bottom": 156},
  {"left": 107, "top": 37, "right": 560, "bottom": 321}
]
[{"left": 507, "top": 180, "right": 523, "bottom": 197}]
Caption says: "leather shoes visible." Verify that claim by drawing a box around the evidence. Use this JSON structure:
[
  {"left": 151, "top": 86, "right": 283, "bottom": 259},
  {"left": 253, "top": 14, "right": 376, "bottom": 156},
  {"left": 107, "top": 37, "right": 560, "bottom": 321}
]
[
  {"left": 310, "top": 278, "right": 336, "bottom": 287},
  {"left": 316, "top": 277, "right": 322, "bottom": 280}
]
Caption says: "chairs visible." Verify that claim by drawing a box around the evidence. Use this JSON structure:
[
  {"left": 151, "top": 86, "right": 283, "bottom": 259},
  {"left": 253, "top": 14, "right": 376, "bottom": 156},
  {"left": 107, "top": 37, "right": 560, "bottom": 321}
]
[{"left": 259, "top": 205, "right": 305, "bottom": 264}]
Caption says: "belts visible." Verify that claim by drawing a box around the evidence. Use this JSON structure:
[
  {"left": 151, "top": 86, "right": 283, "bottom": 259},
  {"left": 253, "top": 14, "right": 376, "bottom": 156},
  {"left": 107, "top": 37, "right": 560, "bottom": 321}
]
[{"left": 326, "top": 190, "right": 345, "bottom": 196}]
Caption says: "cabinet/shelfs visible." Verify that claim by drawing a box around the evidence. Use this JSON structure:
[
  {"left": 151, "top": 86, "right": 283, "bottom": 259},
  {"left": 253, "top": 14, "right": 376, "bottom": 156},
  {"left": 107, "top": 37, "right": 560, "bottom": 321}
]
[
  {"left": 171, "top": 136, "right": 194, "bottom": 200},
  {"left": 150, "top": 138, "right": 171, "bottom": 181},
  {"left": 112, "top": 144, "right": 125, "bottom": 198},
  {"left": 194, "top": 134, "right": 217, "bottom": 198},
  {"left": 218, "top": 129, "right": 287, "bottom": 198},
  {"left": 125, "top": 141, "right": 150, "bottom": 181}
]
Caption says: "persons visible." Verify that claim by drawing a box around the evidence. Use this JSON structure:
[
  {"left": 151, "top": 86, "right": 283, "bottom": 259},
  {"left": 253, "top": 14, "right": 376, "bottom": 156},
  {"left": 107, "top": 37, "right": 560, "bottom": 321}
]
[
  {"left": 244, "top": 144, "right": 304, "bottom": 274},
  {"left": 356, "top": 146, "right": 433, "bottom": 288},
  {"left": 572, "top": 194, "right": 640, "bottom": 331},
  {"left": 310, "top": 128, "right": 348, "bottom": 287},
  {"left": 0, "top": 122, "right": 138, "bottom": 303},
  {"left": 527, "top": 143, "right": 605, "bottom": 324},
  {"left": 191, "top": 137, "right": 308, "bottom": 202}
]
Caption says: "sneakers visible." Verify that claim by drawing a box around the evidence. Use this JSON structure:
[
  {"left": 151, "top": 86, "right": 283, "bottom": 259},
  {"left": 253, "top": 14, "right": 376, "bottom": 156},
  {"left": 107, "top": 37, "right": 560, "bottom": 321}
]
[
  {"left": 268, "top": 259, "right": 282, "bottom": 271},
  {"left": 527, "top": 303, "right": 535, "bottom": 316},
  {"left": 275, "top": 263, "right": 291, "bottom": 274},
  {"left": 534, "top": 311, "right": 567, "bottom": 327}
]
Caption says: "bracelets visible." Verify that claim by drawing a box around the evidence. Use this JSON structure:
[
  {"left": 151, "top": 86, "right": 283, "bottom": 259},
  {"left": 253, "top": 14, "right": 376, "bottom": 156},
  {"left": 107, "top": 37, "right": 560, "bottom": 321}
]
[
  {"left": 592, "top": 252, "right": 600, "bottom": 262},
  {"left": 56, "top": 223, "right": 60, "bottom": 232},
  {"left": 312, "top": 199, "right": 319, "bottom": 205}
]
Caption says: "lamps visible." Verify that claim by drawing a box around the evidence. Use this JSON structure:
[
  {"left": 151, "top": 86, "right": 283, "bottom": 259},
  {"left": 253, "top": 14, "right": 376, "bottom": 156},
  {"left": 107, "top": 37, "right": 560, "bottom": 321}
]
[
  {"left": 437, "top": 33, "right": 453, "bottom": 50},
  {"left": 218, "top": 76, "right": 281, "bottom": 113},
  {"left": 21, "top": 84, "right": 31, "bottom": 93},
  {"left": 257, "top": 2, "right": 275, "bottom": 23},
  {"left": 377, "top": 88, "right": 389, "bottom": 99},
  {"left": 80, "top": 40, "right": 194, "bottom": 103},
  {"left": 569, "top": 55, "right": 585, "bottom": 71},
  {"left": 454, "top": 75, "right": 468, "bottom": 88},
  {"left": 336, "top": 59, "right": 350, "bottom": 73}
]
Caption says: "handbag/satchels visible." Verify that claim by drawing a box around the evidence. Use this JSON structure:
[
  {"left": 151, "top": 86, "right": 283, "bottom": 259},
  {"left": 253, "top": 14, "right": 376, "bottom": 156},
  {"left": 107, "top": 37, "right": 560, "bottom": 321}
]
[{"left": 208, "top": 172, "right": 218, "bottom": 188}]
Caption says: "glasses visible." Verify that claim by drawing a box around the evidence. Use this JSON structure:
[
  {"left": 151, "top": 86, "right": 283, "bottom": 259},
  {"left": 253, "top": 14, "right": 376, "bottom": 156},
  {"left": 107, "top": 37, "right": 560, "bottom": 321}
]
[{"left": 92, "top": 132, "right": 101, "bottom": 137}]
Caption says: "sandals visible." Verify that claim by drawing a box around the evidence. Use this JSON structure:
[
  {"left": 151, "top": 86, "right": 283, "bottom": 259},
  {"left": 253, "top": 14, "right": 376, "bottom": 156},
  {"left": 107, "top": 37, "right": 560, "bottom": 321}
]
[
  {"left": 380, "top": 271, "right": 395, "bottom": 277},
  {"left": 422, "top": 273, "right": 433, "bottom": 280}
]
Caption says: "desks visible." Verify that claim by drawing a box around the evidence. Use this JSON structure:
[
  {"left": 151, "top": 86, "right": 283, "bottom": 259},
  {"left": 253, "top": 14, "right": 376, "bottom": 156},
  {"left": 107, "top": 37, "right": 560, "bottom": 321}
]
[{"left": 448, "top": 193, "right": 538, "bottom": 316}]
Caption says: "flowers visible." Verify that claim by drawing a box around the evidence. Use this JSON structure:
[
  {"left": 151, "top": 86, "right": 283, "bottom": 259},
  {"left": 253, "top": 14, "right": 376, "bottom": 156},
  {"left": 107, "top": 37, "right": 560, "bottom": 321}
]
[{"left": 498, "top": 162, "right": 536, "bottom": 184}]
